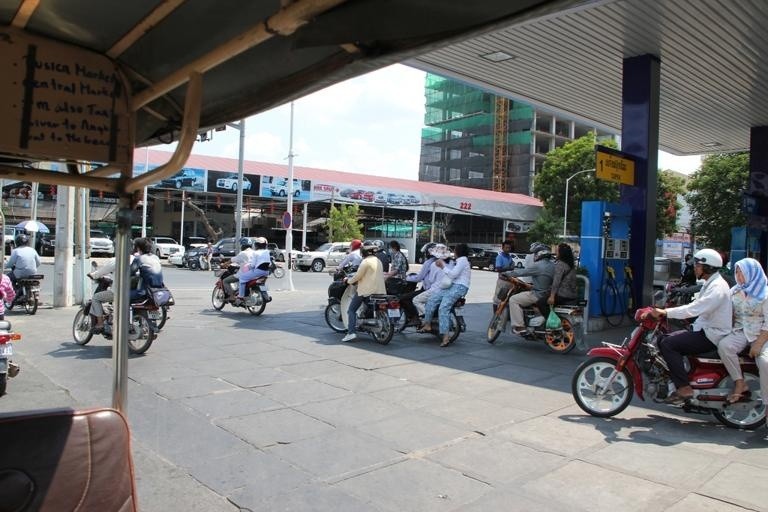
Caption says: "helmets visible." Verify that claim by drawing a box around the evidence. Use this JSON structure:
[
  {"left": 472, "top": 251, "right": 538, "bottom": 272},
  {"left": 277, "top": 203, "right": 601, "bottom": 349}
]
[
  {"left": 254, "top": 236, "right": 268, "bottom": 244},
  {"left": 350, "top": 239, "right": 362, "bottom": 251},
  {"left": 359, "top": 240, "right": 379, "bottom": 256},
  {"left": 533, "top": 250, "right": 551, "bottom": 262},
  {"left": 685, "top": 248, "right": 723, "bottom": 268},
  {"left": 421, "top": 243, "right": 436, "bottom": 259},
  {"left": 15, "top": 234, "right": 29, "bottom": 245},
  {"left": 239, "top": 237, "right": 253, "bottom": 247},
  {"left": 715, "top": 249, "right": 728, "bottom": 266},
  {"left": 373, "top": 239, "right": 384, "bottom": 251}
]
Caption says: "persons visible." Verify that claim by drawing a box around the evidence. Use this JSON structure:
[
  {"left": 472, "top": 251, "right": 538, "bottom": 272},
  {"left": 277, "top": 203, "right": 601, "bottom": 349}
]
[
  {"left": 234, "top": 237, "right": 271, "bottom": 301},
  {"left": 374, "top": 240, "right": 388, "bottom": 273},
  {"left": 651, "top": 248, "right": 731, "bottom": 404},
  {"left": 3, "top": 234, "right": 40, "bottom": 298},
  {"left": 338, "top": 239, "right": 364, "bottom": 275},
  {"left": 1, "top": 270, "right": 15, "bottom": 321},
  {"left": 502, "top": 242, "right": 555, "bottom": 335},
  {"left": 384, "top": 241, "right": 408, "bottom": 295},
  {"left": 680, "top": 254, "right": 696, "bottom": 304},
  {"left": 719, "top": 258, "right": 768, "bottom": 428},
  {"left": 400, "top": 243, "right": 437, "bottom": 328},
  {"left": 493, "top": 242, "right": 515, "bottom": 320},
  {"left": 412, "top": 243, "right": 455, "bottom": 330},
  {"left": 341, "top": 240, "right": 386, "bottom": 342},
  {"left": 108, "top": 238, "right": 163, "bottom": 325},
  {"left": 537, "top": 244, "right": 578, "bottom": 331},
  {"left": 203, "top": 242, "right": 213, "bottom": 272},
  {"left": 417, "top": 244, "right": 470, "bottom": 346},
  {"left": 89, "top": 236, "right": 139, "bottom": 336},
  {"left": 670, "top": 249, "right": 737, "bottom": 295},
  {"left": 223, "top": 237, "right": 255, "bottom": 302}
]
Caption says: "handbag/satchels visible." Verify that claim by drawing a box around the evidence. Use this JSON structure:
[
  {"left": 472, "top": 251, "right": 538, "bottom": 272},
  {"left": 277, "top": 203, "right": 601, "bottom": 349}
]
[
  {"left": 143, "top": 280, "right": 175, "bottom": 309},
  {"left": 439, "top": 274, "right": 452, "bottom": 289}
]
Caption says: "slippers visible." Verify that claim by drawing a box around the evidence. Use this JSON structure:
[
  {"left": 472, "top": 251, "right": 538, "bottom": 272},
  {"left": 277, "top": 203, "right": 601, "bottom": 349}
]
[
  {"left": 725, "top": 390, "right": 753, "bottom": 404},
  {"left": 663, "top": 391, "right": 694, "bottom": 404},
  {"left": 416, "top": 328, "right": 432, "bottom": 333},
  {"left": 440, "top": 336, "right": 452, "bottom": 347}
]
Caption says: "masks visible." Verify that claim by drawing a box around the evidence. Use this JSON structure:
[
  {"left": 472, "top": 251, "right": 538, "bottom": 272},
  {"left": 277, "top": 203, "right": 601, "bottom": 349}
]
[{"left": 388, "top": 248, "right": 395, "bottom": 254}]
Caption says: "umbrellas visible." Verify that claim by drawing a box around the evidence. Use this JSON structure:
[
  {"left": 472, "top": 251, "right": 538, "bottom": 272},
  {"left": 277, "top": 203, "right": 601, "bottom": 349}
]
[{"left": 15, "top": 221, "right": 50, "bottom": 233}]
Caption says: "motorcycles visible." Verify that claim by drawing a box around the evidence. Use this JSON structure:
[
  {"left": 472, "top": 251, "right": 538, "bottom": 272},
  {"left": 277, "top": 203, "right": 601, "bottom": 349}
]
[
  {"left": 2, "top": 256, "right": 45, "bottom": 316},
  {"left": 270, "top": 256, "right": 286, "bottom": 280},
  {"left": 0, "top": 312, "right": 24, "bottom": 395},
  {"left": 324, "top": 263, "right": 398, "bottom": 342},
  {"left": 152, "top": 304, "right": 172, "bottom": 332},
  {"left": 71, "top": 260, "right": 158, "bottom": 355},
  {"left": 395, "top": 272, "right": 465, "bottom": 344},
  {"left": 483, "top": 270, "right": 585, "bottom": 352},
  {"left": 572, "top": 301, "right": 764, "bottom": 431},
  {"left": 210, "top": 261, "right": 273, "bottom": 314},
  {"left": 658, "top": 282, "right": 685, "bottom": 307},
  {"left": 391, "top": 279, "right": 411, "bottom": 334}
]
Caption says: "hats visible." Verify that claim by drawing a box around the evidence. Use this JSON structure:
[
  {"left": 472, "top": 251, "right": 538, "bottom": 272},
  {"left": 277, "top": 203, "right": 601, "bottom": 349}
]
[{"left": 428, "top": 243, "right": 455, "bottom": 260}]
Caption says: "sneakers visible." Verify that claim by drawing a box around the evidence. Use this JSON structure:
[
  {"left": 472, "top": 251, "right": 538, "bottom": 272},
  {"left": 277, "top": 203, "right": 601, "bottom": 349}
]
[
  {"left": 512, "top": 328, "right": 527, "bottom": 335},
  {"left": 341, "top": 333, "right": 357, "bottom": 342}
]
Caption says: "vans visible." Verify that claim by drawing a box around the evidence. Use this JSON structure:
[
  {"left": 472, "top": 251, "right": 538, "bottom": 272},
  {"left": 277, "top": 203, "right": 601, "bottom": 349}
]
[
  {"left": 185, "top": 236, "right": 208, "bottom": 249},
  {"left": 388, "top": 192, "right": 402, "bottom": 205},
  {"left": 340, "top": 188, "right": 354, "bottom": 198},
  {"left": 35, "top": 227, "right": 56, "bottom": 257},
  {"left": 360, "top": 190, "right": 375, "bottom": 201},
  {"left": 399, "top": 193, "right": 410, "bottom": 204},
  {"left": 383, "top": 240, "right": 410, "bottom": 261},
  {"left": 350, "top": 189, "right": 366, "bottom": 200},
  {"left": 0, "top": 223, "right": 19, "bottom": 254},
  {"left": 269, "top": 180, "right": 302, "bottom": 197},
  {"left": 409, "top": 194, "right": 420, "bottom": 204},
  {"left": 374, "top": 190, "right": 387, "bottom": 203},
  {"left": 183, "top": 235, "right": 260, "bottom": 272}
]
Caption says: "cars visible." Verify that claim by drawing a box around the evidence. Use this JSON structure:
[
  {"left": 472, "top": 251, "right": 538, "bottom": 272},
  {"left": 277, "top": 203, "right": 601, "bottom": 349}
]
[
  {"left": 274, "top": 246, "right": 302, "bottom": 262},
  {"left": 147, "top": 235, "right": 185, "bottom": 258},
  {"left": 510, "top": 253, "right": 526, "bottom": 269},
  {"left": 214, "top": 173, "right": 254, "bottom": 191},
  {"left": 161, "top": 169, "right": 200, "bottom": 188},
  {"left": 468, "top": 248, "right": 498, "bottom": 271}
]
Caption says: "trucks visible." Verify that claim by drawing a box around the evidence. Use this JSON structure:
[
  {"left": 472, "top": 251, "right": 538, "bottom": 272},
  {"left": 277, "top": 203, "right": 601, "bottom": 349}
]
[{"left": 385, "top": 240, "right": 409, "bottom": 263}]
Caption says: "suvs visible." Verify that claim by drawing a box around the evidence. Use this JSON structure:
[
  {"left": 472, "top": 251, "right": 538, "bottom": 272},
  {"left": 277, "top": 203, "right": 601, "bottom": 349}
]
[
  {"left": 294, "top": 241, "right": 362, "bottom": 271},
  {"left": 88, "top": 228, "right": 115, "bottom": 258}
]
